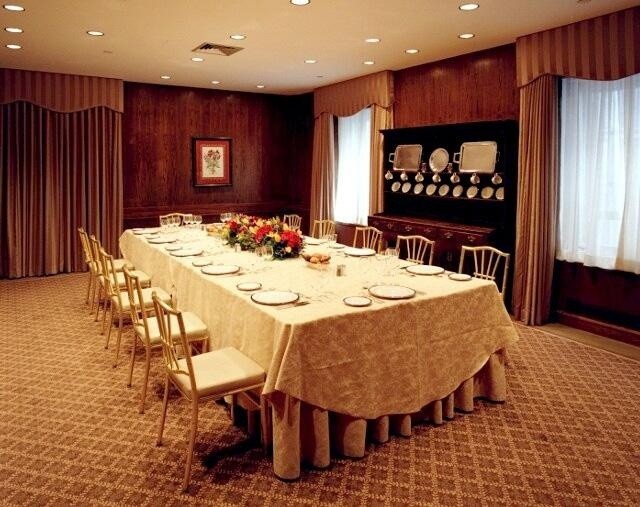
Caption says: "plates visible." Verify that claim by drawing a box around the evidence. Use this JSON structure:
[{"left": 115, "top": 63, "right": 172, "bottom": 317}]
[
  {"left": 342, "top": 296, "right": 372, "bottom": 307},
  {"left": 133, "top": 226, "right": 300, "bottom": 305},
  {"left": 302, "top": 238, "right": 472, "bottom": 280},
  {"left": 391, "top": 181, "right": 505, "bottom": 202},
  {"left": 428, "top": 148, "right": 449, "bottom": 173},
  {"left": 369, "top": 284, "right": 416, "bottom": 299}
]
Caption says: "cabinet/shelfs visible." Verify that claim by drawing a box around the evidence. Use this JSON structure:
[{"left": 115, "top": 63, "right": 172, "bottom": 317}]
[{"left": 385, "top": 170, "right": 505, "bottom": 204}]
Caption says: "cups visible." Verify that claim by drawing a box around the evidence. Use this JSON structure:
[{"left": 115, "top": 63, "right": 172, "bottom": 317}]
[
  {"left": 421, "top": 163, "right": 427, "bottom": 173},
  {"left": 447, "top": 163, "right": 453, "bottom": 174},
  {"left": 384, "top": 170, "right": 503, "bottom": 185}
]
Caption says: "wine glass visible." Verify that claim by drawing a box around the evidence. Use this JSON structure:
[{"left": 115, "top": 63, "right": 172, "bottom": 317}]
[
  {"left": 159, "top": 213, "right": 202, "bottom": 232},
  {"left": 220, "top": 213, "right": 231, "bottom": 225}
]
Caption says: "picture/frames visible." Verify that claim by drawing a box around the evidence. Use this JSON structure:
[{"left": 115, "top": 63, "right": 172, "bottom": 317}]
[{"left": 193, "top": 137, "right": 233, "bottom": 188}]
